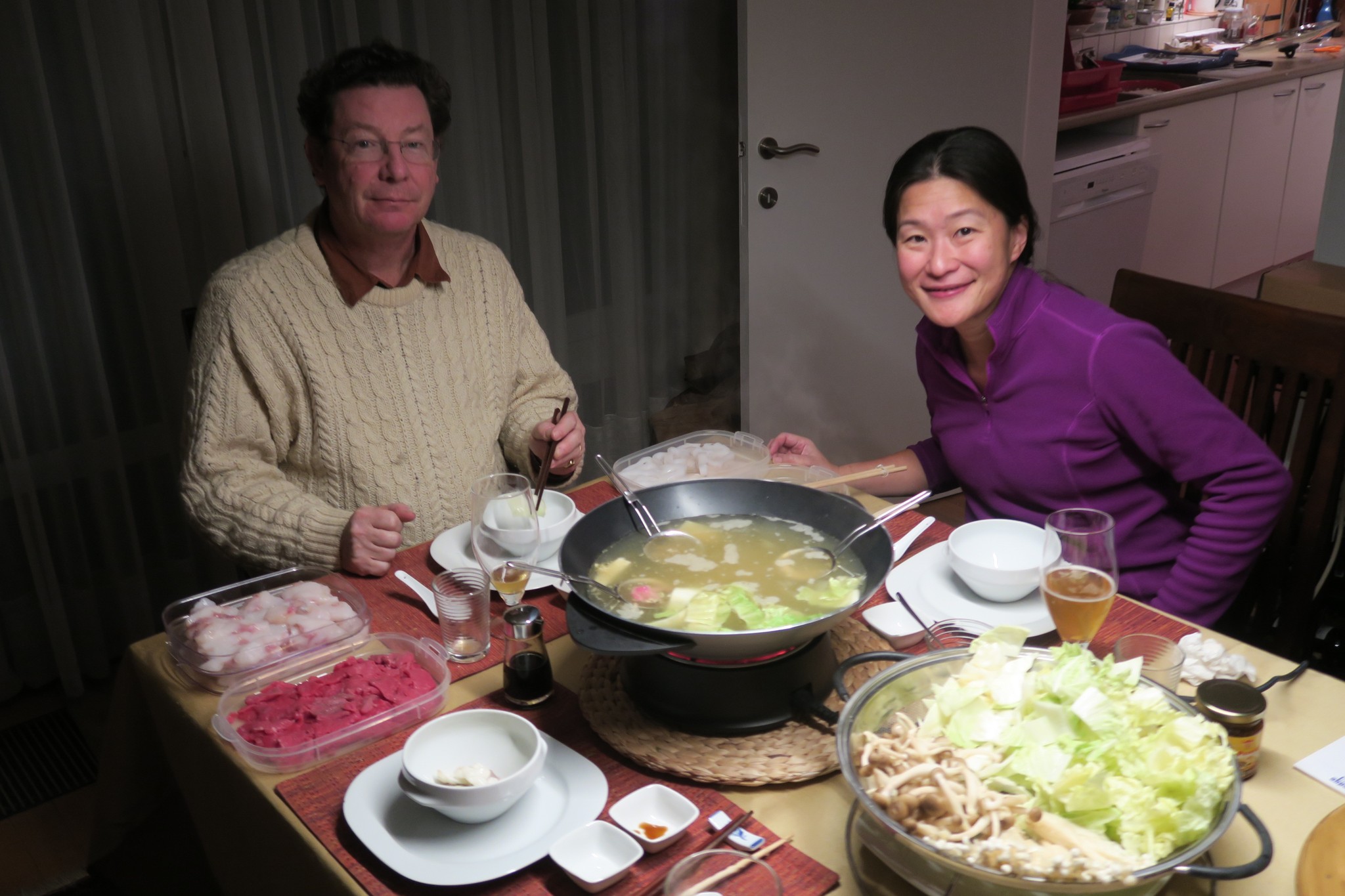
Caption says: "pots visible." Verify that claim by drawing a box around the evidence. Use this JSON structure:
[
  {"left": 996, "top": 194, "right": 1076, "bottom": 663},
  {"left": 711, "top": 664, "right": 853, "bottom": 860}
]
[
  {"left": 829, "top": 642, "right": 1272, "bottom": 896},
  {"left": 535, "top": 476, "right": 893, "bottom": 661}
]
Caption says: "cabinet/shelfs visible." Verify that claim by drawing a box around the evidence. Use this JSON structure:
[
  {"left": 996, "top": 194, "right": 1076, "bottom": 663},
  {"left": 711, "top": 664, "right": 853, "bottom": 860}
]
[
  {"left": 1135, "top": 92, "right": 1235, "bottom": 290},
  {"left": 1210, "top": 67, "right": 1345, "bottom": 291}
]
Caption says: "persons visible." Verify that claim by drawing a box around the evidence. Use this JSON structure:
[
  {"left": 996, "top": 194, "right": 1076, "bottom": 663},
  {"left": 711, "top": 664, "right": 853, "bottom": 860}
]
[
  {"left": 767, "top": 126, "right": 1293, "bottom": 626},
  {"left": 178, "top": 44, "right": 586, "bottom": 579}
]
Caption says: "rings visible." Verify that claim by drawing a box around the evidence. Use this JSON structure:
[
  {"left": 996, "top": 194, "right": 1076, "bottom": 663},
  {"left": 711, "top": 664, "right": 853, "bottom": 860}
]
[
  {"left": 564, "top": 460, "right": 576, "bottom": 468},
  {"left": 579, "top": 444, "right": 583, "bottom": 452}
]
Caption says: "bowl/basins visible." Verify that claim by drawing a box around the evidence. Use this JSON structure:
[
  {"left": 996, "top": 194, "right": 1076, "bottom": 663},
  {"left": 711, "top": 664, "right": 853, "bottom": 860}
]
[
  {"left": 399, "top": 708, "right": 548, "bottom": 825},
  {"left": 665, "top": 849, "right": 783, "bottom": 896},
  {"left": 610, "top": 783, "right": 699, "bottom": 849},
  {"left": 924, "top": 617, "right": 997, "bottom": 650},
  {"left": 160, "top": 566, "right": 373, "bottom": 694},
  {"left": 947, "top": 518, "right": 1062, "bottom": 600},
  {"left": 214, "top": 632, "right": 453, "bottom": 772},
  {"left": 612, "top": 430, "right": 773, "bottom": 495},
  {"left": 483, "top": 485, "right": 576, "bottom": 562},
  {"left": 861, "top": 599, "right": 936, "bottom": 649},
  {"left": 548, "top": 819, "right": 644, "bottom": 891}
]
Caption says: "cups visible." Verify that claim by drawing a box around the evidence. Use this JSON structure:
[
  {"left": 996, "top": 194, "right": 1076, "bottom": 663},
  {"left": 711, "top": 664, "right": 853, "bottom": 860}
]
[
  {"left": 432, "top": 565, "right": 491, "bottom": 663},
  {"left": 1114, "top": 633, "right": 1186, "bottom": 692}
]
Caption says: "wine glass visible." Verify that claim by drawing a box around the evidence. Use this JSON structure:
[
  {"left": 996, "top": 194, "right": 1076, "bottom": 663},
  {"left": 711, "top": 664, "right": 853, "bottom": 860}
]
[
  {"left": 1040, "top": 507, "right": 1119, "bottom": 652},
  {"left": 471, "top": 472, "right": 541, "bottom": 642}
]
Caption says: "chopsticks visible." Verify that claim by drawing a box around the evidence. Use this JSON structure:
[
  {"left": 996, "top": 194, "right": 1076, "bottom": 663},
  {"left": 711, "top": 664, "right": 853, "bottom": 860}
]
[
  {"left": 535, "top": 397, "right": 570, "bottom": 512},
  {"left": 631, "top": 812, "right": 752, "bottom": 896},
  {"left": 802, "top": 462, "right": 908, "bottom": 489}
]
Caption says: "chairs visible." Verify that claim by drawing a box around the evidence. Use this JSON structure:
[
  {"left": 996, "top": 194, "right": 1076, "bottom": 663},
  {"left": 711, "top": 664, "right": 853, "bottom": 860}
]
[{"left": 1111, "top": 268, "right": 1345, "bottom": 644}]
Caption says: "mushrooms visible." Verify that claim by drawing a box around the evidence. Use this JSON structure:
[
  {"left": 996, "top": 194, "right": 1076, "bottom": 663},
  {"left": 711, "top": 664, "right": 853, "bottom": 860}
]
[{"left": 846, "top": 712, "right": 1150, "bottom": 885}]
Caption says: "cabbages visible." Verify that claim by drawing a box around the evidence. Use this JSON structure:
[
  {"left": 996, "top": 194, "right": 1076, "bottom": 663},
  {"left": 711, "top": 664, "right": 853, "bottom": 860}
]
[{"left": 916, "top": 610, "right": 1235, "bottom": 857}]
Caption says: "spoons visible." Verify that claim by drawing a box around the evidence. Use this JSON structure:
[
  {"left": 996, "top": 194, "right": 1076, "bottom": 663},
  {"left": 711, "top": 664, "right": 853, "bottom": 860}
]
[
  {"left": 897, "top": 592, "right": 947, "bottom": 651},
  {"left": 395, "top": 571, "right": 472, "bottom": 624}
]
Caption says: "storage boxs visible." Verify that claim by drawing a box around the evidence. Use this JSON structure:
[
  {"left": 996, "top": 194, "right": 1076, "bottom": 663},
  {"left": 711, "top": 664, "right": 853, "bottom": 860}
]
[
  {"left": 1137, "top": 10, "right": 1164, "bottom": 25},
  {"left": 1091, "top": 7, "right": 1110, "bottom": 21},
  {"left": 1061, "top": 59, "right": 1126, "bottom": 90},
  {"left": 1086, "top": 19, "right": 1108, "bottom": 33},
  {"left": 1059, "top": 90, "right": 1122, "bottom": 114}
]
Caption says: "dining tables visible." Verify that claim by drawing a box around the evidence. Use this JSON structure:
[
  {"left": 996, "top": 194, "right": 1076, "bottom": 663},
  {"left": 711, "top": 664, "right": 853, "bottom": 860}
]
[{"left": 129, "top": 473, "right": 1344, "bottom": 896}]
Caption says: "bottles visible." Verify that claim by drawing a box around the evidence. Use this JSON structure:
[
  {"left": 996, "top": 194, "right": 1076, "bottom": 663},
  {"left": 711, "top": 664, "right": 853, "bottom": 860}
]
[{"left": 501, "top": 604, "right": 556, "bottom": 708}]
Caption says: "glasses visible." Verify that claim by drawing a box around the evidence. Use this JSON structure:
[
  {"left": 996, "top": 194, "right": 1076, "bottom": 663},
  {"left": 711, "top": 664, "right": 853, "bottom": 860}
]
[{"left": 331, "top": 135, "right": 442, "bottom": 167}]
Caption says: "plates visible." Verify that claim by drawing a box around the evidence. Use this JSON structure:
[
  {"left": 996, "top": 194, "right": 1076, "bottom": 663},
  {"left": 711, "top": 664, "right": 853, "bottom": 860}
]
[
  {"left": 883, "top": 539, "right": 1075, "bottom": 639},
  {"left": 341, "top": 723, "right": 609, "bottom": 884}
]
